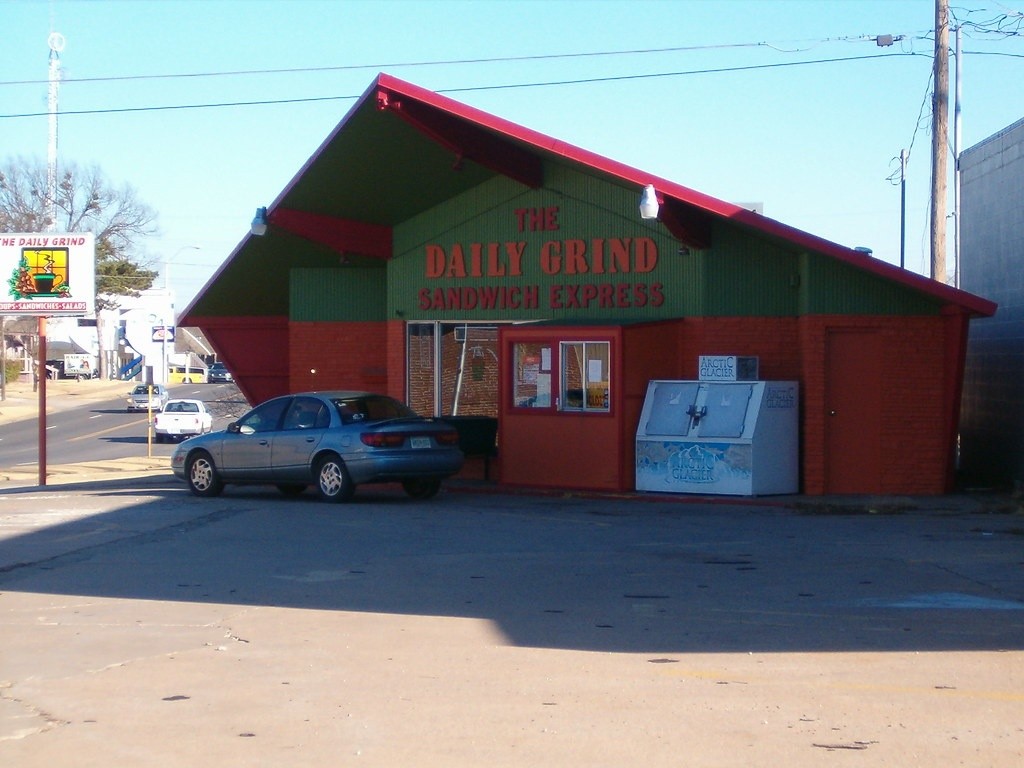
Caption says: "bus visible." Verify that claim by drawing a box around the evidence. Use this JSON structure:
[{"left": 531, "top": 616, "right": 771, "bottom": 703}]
[{"left": 166, "top": 367, "right": 206, "bottom": 385}]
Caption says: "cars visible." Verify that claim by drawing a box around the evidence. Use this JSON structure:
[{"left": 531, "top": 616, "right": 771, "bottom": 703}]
[
  {"left": 208, "top": 362, "right": 235, "bottom": 383},
  {"left": 171, "top": 390, "right": 466, "bottom": 503},
  {"left": 127, "top": 384, "right": 169, "bottom": 413}
]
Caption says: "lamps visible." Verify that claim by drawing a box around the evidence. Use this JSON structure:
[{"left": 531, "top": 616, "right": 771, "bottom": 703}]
[{"left": 639, "top": 186, "right": 659, "bottom": 219}]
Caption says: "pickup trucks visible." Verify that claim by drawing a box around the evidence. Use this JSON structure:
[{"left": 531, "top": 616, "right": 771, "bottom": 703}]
[{"left": 154, "top": 398, "right": 212, "bottom": 443}]
